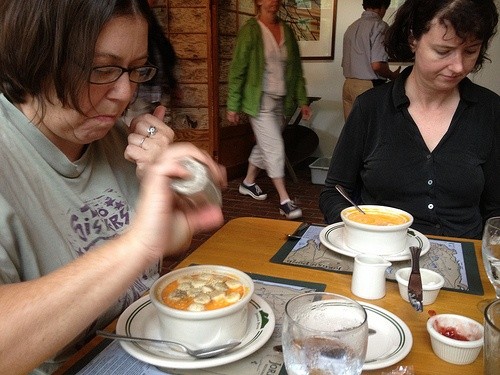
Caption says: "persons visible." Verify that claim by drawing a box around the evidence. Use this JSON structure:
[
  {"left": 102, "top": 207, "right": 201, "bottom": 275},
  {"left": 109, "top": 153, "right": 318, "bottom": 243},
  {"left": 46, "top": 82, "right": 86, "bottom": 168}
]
[
  {"left": 226, "top": 0, "right": 312, "bottom": 218},
  {"left": 320, "top": 0, "right": 500, "bottom": 241},
  {"left": 0, "top": 0, "right": 227, "bottom": 375},
  {"left": 341, "top": 0, "right": 400, "bottom": 123}
]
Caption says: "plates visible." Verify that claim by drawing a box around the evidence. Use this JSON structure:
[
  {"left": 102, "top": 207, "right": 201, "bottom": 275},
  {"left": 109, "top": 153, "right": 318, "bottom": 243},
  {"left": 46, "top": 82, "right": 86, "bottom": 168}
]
[
  {"left": 116, "top": 291, "right": 275, "bottom": 369},
  {"left": 285, "top": 298, "right": 413, "bottom": 370},
  {"left": 319, "top": 222, "right": 430, "bottom": 261}
]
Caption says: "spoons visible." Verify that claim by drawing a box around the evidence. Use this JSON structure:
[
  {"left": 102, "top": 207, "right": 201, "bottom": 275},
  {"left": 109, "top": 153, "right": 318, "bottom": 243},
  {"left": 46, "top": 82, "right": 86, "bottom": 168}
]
[
  {"left": 284, "top": 222, "right": 312, "bottom": 239},
  {"left": 95, "top": 330, "right": 242, "bottom": 360}
]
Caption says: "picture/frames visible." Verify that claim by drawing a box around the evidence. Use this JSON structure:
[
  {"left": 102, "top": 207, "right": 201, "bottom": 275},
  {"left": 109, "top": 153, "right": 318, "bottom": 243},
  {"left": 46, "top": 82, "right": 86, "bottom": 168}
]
[{"left": 280, "top": 0, "right": 338, "bottom": 60}]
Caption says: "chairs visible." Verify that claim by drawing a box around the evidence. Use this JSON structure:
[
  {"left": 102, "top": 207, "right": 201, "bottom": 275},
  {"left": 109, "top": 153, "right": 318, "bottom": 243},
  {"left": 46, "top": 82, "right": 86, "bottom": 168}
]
[{"left": 282, "top": 98, "right": 322, "bottom": 184}]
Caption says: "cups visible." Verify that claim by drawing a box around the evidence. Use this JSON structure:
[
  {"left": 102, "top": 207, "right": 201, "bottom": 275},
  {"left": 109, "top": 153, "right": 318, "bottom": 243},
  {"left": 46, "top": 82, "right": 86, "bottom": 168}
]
[
  {"left": 395, "top": 267, "right": 445, "bottom": 305},
  {"left": 427, "top": 314, "right": 485, "bottom": 365},
  {"left": 281, "top": 292, "right": 369, "bottom": 375},
  {"left": 149, "top": 264, "right": 255, "bottom": 352},
  {"left": 340, "top": 204, "right": 414, "bottom": 255},
  {"left": 484, "top": 298, "right": 500, "bottom": 375},
  {"left": 168, "top": 156, "right": 222, "bottom": 214}
]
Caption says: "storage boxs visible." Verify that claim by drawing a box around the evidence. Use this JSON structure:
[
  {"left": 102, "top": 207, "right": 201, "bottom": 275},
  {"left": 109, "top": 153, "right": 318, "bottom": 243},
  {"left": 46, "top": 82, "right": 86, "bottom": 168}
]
[{"left": 308, "top": 158, "right": 329, "bottom": 184}]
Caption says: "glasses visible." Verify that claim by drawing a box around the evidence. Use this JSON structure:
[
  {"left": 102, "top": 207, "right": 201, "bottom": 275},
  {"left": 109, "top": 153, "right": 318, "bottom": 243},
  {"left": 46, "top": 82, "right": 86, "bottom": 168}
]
[{"left": 74, "top": 60, "right": 158, "bottom": 84}]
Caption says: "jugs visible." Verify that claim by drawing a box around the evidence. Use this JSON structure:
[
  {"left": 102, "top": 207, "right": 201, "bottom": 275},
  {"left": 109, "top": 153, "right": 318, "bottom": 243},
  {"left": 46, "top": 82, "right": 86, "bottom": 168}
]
[{"left": 351, "top": 254, "right": 392, "bottom": 300}]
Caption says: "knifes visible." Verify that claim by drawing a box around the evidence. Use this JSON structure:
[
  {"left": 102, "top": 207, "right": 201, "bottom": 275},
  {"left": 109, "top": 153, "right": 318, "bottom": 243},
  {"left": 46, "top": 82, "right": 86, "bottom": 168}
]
[{"left": 407, "top": 245, "right": 424, "bottom": 312}]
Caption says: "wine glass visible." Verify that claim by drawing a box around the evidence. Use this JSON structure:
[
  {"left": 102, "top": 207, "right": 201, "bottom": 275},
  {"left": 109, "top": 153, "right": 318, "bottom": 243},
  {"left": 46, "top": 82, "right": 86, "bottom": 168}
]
[{"left": 482, "top": 217, "right": 500, "bottom": 314}]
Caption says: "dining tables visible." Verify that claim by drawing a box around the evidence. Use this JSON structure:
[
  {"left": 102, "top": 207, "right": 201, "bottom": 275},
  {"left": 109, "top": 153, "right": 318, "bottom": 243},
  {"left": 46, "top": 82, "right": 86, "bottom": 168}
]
[{"left": 52, "top": 218, "right": 500, "bottom": 375}]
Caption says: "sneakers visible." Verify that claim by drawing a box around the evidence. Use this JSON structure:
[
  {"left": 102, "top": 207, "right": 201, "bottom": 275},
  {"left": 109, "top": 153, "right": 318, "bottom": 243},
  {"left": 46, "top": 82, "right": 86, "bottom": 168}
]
[
  {"left": 279, "top": 201, "right": 302, "bottom": 219},
  {"left": 239, "top": 181, "right": 268, "bottom": 200}
]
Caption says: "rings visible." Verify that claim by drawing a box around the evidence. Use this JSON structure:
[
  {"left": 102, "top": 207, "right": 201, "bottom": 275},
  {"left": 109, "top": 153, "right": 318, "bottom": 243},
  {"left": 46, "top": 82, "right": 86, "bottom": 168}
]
[
  {"left": 148, "top": 126, "right": 156, "bottom": 137},
  {"left": 139, "top": 136, "right": 147, "bottom": 148}
]
